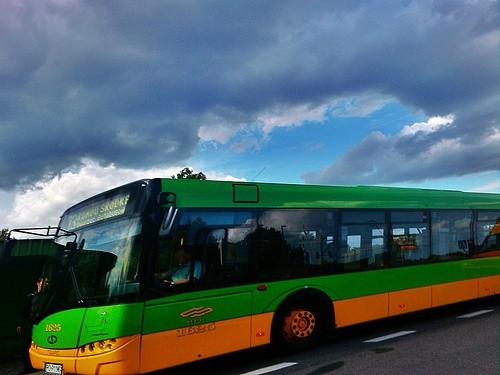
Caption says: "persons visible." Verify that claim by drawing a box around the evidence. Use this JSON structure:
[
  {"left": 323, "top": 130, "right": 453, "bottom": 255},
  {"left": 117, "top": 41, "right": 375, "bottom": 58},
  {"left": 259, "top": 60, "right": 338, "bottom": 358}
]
[
  {"left": 16, "top": 274, "right": 48, "bottom": 372},
  {"left": 155, "top": 248, "right": 203, "bottom": 286}
]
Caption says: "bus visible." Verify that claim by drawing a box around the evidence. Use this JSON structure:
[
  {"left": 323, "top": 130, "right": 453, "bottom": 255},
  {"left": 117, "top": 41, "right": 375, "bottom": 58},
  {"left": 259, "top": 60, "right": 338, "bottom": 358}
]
[{"left": 0, "top": 177, "right": 500, "bottom": 375}]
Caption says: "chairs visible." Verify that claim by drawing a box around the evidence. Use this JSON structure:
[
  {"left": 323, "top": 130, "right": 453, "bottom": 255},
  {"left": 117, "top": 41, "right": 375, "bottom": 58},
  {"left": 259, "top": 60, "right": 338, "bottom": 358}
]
[{"left": 226, "top": 240, "right": 248, "bottom": 273}]
